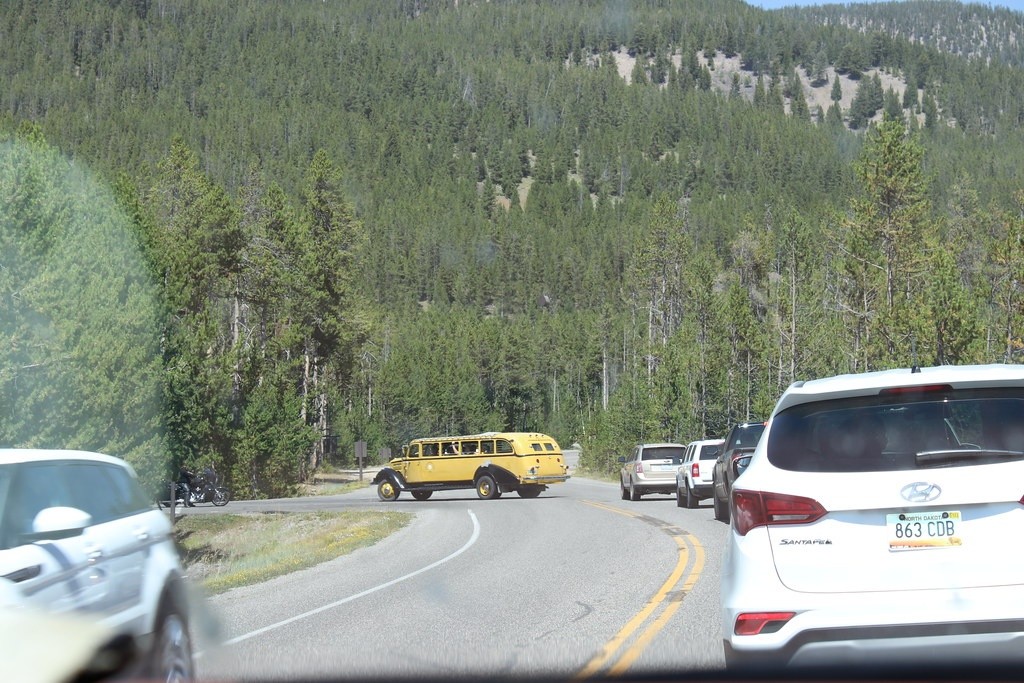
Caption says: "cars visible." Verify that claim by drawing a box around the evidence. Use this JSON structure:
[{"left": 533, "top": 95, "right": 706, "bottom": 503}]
[
  {"left": 617, "top": 442, "right": 687, "bottom": 501},
  {"left": 676, "top": 439, "right": 726, "bottom": 509}
]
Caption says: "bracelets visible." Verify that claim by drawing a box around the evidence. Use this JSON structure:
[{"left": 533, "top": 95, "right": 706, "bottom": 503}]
[{"left": 452, "top": 443, "right": 455, "bottom": 445}]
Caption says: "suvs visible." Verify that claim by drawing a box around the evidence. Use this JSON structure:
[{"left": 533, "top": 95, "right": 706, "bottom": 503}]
[
  {"left": 712, "top": 422, "right": 767, "bottom": 519},
  {"left": 0, "top": 447, "right": 222, "bottom": 683},
  {"left": 722, "top": 362, "right": 1023, "bottom": 674}
]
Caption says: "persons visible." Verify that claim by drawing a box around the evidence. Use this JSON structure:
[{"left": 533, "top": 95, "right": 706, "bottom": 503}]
[
  {"left": 450, "top": 441, "right": 477, "bottom": 454},
  {"left": 178, "top": 463, "right": 196, "bottom": 508}
]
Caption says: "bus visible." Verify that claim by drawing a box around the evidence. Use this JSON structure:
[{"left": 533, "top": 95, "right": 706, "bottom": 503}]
[{"left": 370, "top": 432, "right": 568, "bottom": 502}]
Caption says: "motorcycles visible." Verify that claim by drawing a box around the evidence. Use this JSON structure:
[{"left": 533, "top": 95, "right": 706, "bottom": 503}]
[{"left": 161, "top": 468, "right": 231, "bottom": 507}]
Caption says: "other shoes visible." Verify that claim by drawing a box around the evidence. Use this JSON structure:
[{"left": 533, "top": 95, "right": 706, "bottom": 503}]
[
  {"left": 190, "top": 504, "right": 195, "bottom": 507},
  {"left": 184, "top": 505, "right": 190, "bottom": 508}
]
